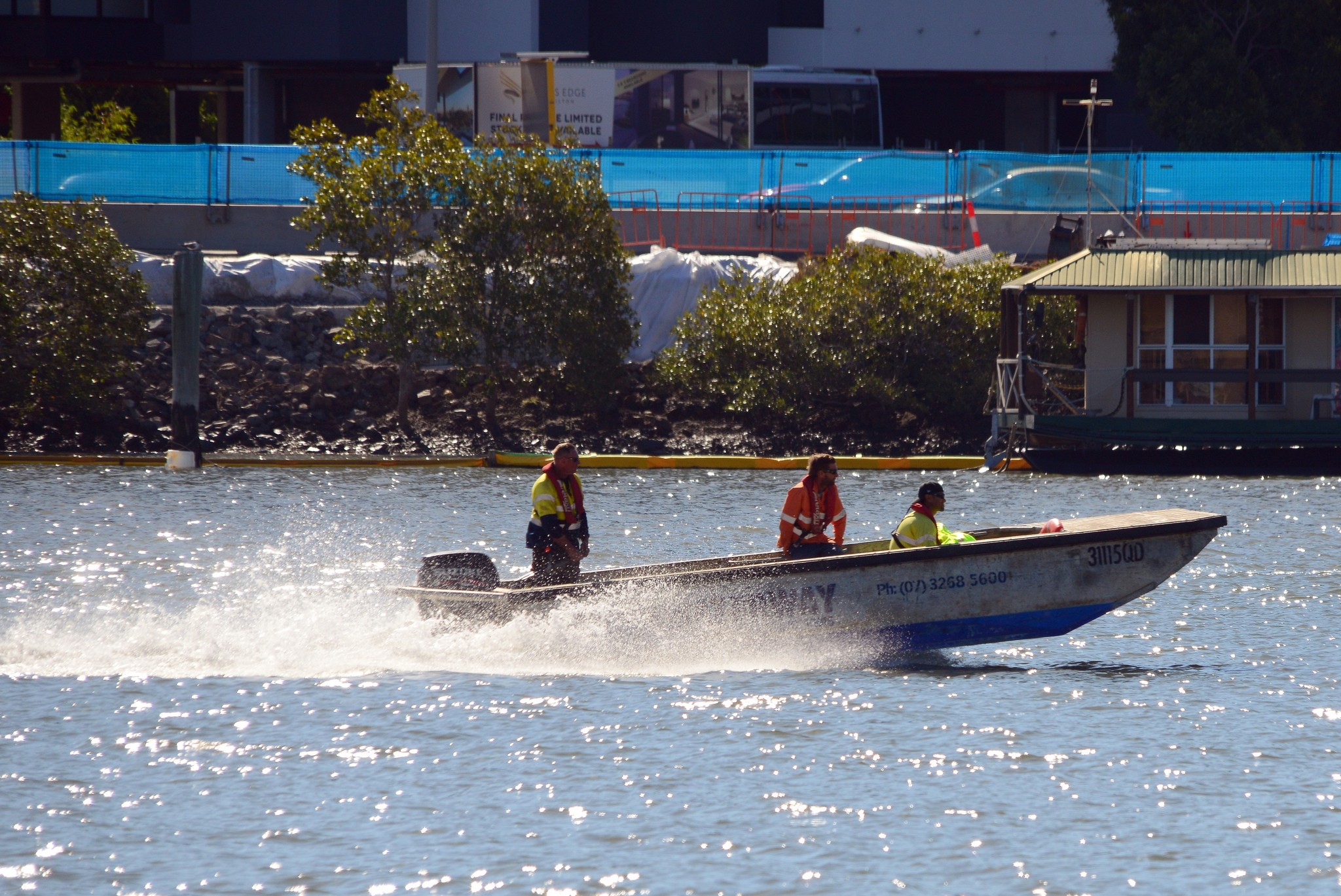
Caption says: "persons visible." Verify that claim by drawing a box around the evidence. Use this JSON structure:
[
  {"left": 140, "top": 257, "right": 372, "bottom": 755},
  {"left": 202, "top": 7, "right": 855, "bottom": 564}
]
[
  {"left": 888, "top": 481, "right": 976, "bottom": 551},
  {"left": 777, "top": 453, "right": 846, "bottom": 560},
  {"left": 525, "top": 444, "right": 590, "bottom": 586}
]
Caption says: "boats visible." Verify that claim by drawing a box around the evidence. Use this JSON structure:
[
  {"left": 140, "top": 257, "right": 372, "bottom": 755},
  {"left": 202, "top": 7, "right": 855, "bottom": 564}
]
[{"left": 384, "top": 507, "right": 1233, "bottom": 659}]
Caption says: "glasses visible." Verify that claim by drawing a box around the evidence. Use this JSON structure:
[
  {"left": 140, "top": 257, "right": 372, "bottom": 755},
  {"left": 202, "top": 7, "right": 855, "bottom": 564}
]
[
  {"left": 823, "top": 469, "right": 837, "bottom": 475},
  {"left": 565, "top": 456, "right": 579, "bottom": 462},
  {"left": 933, "top": 494, "right": 945, "bottom": 499}
]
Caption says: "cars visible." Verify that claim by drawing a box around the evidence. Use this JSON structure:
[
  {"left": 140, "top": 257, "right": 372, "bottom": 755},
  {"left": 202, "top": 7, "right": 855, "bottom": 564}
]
[
  {"left": 915, "top": 164, "right": 1175, "bottom": 212},
  {"left": 58, "top": 171, "right": 134, "bottom": 192},
  {"left": 733, "top": 150, "right": 963, "bottom": 211}
]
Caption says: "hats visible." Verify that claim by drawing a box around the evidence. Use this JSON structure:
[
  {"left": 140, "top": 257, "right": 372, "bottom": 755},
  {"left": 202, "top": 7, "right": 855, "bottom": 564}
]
[{"left": 918, "top": 481, "right": 944, "bottom": 497}]
